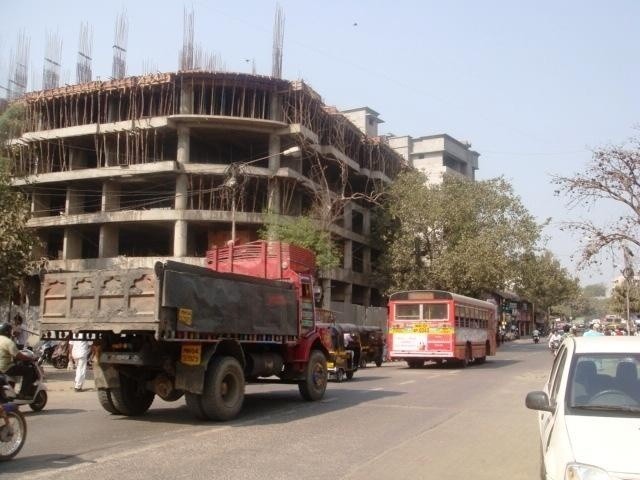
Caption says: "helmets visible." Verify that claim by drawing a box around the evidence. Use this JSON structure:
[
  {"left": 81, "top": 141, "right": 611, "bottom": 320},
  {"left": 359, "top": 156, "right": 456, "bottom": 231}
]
[{"left": 0, "top": 323, "right": 12, "bottom": 332}]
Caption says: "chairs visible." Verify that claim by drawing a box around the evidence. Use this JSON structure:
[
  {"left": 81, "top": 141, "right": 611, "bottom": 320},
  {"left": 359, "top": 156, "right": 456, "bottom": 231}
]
[{"left": 576, "top": 359, "right": 639, "bottom": 393}]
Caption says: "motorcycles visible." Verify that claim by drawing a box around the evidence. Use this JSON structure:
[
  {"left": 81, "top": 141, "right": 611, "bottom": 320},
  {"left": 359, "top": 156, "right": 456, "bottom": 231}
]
[
  {"left": 51, "top": 335, "right": 97, "bottom": 370},
  {"left": 0, "top": 347, "right": 50, "bottom": 414},
  {"left": 532, "top": 335, "right": 540, "bottom": 345},
  {"left": 550, "top": 339, "right": 560, "bottom": 358},
  {"left": 0, "top": 372, "right": 29, "bottom": 464},
  {"left": 354, "top": 323, "right": 385, "bottom": 369},
  {"left": 33, "top": 330, "right": 59, "bottom": 367},
  {"left": 313, "top": 320, "right": 362, "bottom": 382}
]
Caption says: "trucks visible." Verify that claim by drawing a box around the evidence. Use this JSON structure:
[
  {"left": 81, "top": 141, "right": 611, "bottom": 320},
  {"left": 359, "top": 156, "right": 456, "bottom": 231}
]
[{"left": 38, "top": 237, "right": 335, "bottom": 421}]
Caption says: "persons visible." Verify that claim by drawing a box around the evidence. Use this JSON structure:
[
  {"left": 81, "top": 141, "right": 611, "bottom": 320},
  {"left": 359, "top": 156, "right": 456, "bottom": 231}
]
[
  {"left": 0, "top": 323, "right": 39, "bottom": 401},
  {"left": 544, "top": 319, "right": 640, "bottom": 353},
  {"left": 68, "top": 333, "right": 92, "bottom": 392},
  {"left": 13, "top": 314, "right": 26, "bottom": 351},
  {"left": 531, "top": 328, "right": 542, "bottom": 341}
]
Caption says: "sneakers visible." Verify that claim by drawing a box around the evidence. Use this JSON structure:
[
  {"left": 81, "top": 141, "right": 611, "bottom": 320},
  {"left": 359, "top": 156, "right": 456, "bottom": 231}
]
[{"left": 19, "top": 394, "right": 33, "bottom": 400}]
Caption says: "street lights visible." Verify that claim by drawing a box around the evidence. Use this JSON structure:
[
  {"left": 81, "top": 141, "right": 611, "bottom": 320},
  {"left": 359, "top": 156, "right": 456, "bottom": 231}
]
[{"left": 227, "top": 146, "right": 303, "bottom": 245}]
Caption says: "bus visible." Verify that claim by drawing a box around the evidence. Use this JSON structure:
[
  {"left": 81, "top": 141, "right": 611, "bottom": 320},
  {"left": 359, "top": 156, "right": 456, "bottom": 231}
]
[{"left": 386, "top": 288, "right": 498, "bottom": 370}]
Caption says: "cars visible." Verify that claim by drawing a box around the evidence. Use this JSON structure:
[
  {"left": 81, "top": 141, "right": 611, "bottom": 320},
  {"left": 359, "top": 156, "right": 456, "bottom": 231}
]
[{"left": 523, "top": 333, "right": 640, "bottom": 480}]
